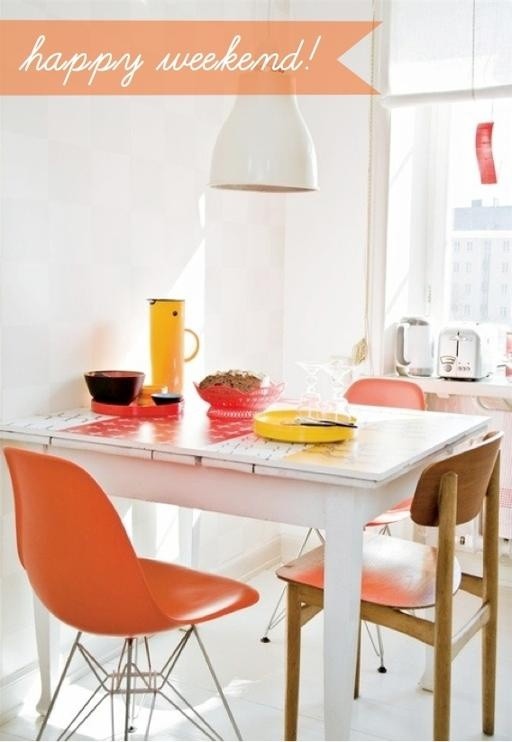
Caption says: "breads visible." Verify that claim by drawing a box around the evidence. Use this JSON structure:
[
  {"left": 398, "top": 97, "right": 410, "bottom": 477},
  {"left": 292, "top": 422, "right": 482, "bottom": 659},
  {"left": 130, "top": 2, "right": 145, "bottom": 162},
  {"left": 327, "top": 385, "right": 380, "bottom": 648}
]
[{"left": 199, "top": 371, "right": 261, "bottom": 393}]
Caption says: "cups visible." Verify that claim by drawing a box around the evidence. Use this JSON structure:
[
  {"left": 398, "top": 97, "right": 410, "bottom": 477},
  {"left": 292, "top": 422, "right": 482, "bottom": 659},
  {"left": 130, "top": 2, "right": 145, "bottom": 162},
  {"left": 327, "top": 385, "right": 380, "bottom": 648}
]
[{"left": 147, "top": 297, "right": 200, "bottom": 393}]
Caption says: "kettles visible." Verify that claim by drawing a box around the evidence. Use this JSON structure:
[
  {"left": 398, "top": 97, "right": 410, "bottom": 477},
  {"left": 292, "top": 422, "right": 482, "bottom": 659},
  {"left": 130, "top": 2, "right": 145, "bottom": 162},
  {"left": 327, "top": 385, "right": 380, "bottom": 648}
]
[{"left": 395, "top": 315, "right": 435, "bottom": 377}]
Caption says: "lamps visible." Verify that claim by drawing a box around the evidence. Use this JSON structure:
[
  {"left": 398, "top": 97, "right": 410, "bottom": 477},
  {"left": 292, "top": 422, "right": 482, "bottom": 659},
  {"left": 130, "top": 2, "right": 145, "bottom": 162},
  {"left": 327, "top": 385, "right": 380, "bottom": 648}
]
[{"left": 209, "top": 1, "right": 319, "bottom": 193}]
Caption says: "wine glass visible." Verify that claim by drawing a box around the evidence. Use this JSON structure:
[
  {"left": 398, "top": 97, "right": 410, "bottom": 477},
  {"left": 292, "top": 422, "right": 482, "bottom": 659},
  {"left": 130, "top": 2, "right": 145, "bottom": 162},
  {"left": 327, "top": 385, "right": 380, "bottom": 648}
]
[{"left": 295, "top": 360, "right": 353, "bottom": 424}]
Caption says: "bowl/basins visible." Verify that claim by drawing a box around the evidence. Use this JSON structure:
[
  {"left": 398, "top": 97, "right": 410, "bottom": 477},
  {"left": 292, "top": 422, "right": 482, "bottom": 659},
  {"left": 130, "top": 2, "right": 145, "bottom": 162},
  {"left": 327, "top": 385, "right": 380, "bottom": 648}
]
[
  {"left": 151, "top": 393, "right": 181, "bottom": 405},
  {"left": 83, "top": 370, "right": 145, "bottom": 406}
]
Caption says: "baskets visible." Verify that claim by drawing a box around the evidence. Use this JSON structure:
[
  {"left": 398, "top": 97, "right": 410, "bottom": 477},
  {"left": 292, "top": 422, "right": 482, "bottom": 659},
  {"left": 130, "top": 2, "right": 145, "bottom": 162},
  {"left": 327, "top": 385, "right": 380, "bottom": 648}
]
[{"left": 193, "top": 380, "right": 284, "bottom": 419}]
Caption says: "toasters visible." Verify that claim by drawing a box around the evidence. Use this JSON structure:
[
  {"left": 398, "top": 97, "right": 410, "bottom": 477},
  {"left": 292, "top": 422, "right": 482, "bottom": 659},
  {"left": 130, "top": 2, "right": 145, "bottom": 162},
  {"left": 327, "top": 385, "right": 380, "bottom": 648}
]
[{"left": 437, "top": 321, "right": 497, "bottom": 383}]
